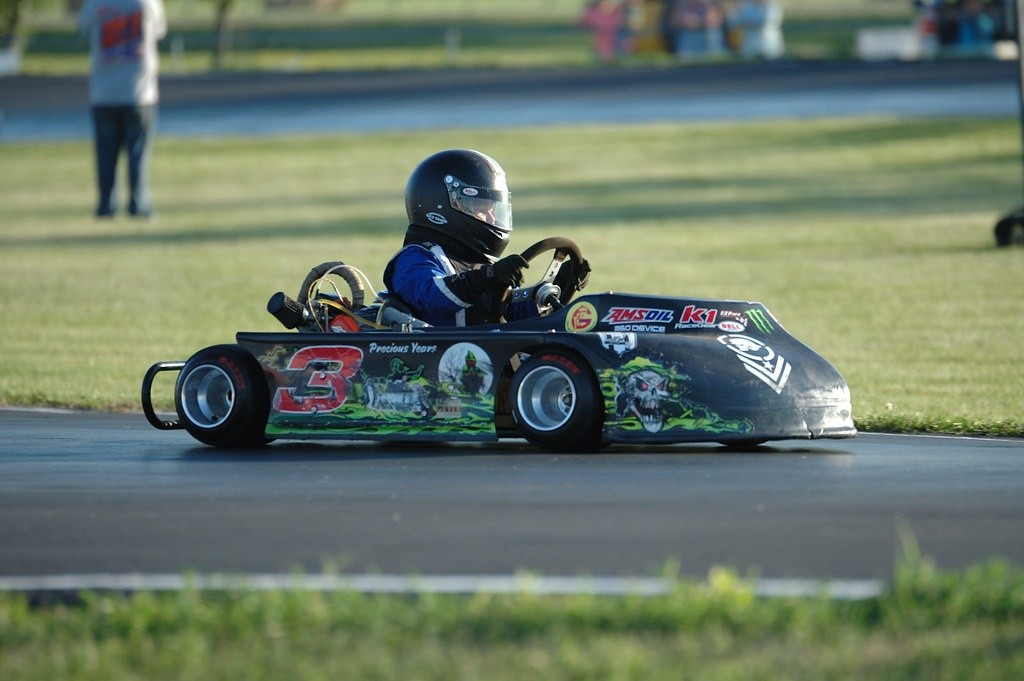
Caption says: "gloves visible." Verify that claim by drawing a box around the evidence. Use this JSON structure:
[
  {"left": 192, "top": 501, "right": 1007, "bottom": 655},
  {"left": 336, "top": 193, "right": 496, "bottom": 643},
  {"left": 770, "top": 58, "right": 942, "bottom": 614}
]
[
  {"left": 479, "top": 254, "right": 529, "bottom": 288},
  {"left": 552, "top": 257, "right": 591, "bottom": 294}
]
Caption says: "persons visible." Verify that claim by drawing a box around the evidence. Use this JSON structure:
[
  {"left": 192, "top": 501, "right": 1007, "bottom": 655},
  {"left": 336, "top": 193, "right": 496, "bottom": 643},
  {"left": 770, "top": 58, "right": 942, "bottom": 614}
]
[
  {"left": 80, "top": 0, "right": 167, "bottom": 219},
  {"left": 382, "top": 148, "right": 592, "bottom": 328},
  {"left": 580, "top": 0, "right": 785, "bottom": 61}
]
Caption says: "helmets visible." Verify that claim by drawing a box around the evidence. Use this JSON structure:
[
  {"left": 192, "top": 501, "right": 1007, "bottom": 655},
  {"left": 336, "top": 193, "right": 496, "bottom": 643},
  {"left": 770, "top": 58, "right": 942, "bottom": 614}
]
[{"left": 404, "top": 148, "right": 512, "bottom": 258}]
